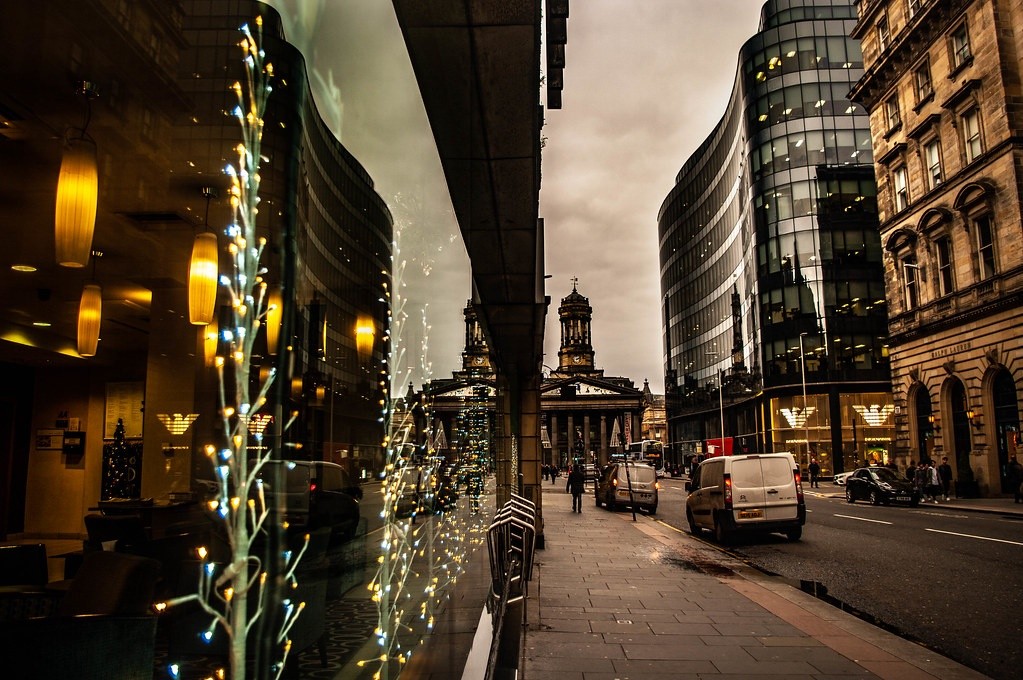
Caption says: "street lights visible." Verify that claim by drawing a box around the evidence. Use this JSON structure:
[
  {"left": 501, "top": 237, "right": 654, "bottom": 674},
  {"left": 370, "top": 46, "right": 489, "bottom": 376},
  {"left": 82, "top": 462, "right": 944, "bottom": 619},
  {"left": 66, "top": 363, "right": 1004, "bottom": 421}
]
[
  {"left": 647, "top": 402, "right": 655, "bottom": 449},
  {"left": 799, "top": 333, "right": 811, "bottom": 484},
  {"left": 705, "top": 352, "right": 725, "bottom": 457}
]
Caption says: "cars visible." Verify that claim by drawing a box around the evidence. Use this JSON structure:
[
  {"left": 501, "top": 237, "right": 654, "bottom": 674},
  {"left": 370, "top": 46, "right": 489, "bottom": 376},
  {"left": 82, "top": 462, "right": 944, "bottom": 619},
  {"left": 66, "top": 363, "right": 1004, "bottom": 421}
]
[
  {"left": 833, "top": 463, "right": 919, "bottom": 507},
  {"left": 666, "top": 464, "right": 680, "bottom": 477},
  {"left": 581, "top": 464, "right": 595, "bottom": 480},
  {"left": 459, "top": 467, "right": 475, "bottom": 483},
  {"left": 375, "top": 471, "right": 390, "bottom": 480}
]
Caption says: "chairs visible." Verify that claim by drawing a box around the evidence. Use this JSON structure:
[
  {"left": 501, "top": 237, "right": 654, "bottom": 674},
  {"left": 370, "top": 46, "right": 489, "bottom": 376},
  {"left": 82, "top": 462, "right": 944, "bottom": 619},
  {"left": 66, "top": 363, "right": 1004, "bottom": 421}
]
[{"left": 0, "top": 543, "right": 50, "bottom": 595}]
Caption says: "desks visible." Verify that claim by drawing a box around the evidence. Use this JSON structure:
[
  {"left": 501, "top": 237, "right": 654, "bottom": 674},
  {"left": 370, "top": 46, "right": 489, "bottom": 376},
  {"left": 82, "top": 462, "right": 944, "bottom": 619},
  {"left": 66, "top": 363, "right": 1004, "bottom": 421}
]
[{"left": 87, "top": 498, "right": 186, "bottom": 530}]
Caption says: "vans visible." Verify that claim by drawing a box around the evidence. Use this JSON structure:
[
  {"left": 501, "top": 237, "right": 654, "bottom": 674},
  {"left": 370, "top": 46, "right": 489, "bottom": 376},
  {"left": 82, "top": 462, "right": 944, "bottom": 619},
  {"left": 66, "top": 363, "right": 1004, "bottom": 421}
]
[
  {"left": 684, "top": 451, "right": 805, "bottom": 548},
  {"left": 249, "top": 457, "right": 361, "bottom": 551},
  {"left": 596, "top": 462, "right": 658, "bottom": 514},
  {"left": 394, "top": 468, "right": 439, "bottom": 515}
]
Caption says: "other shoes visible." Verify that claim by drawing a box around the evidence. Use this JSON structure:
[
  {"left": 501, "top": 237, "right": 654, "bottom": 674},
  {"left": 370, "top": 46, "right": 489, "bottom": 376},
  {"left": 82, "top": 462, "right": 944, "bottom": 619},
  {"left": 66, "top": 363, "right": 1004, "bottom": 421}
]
[
  {"left": 578, "top": 510, "right": 581, "bottom": 513},
  {"left": 920, "top": 498, "right": 926, "bottom": 502},
  {"left": 572, "top": 506, "right": 576, "bottom": 511},
  {"left": 929, "top": 498, "right": 931, "bottom": 500},
  {"left": 811, "top": 486, "right": 813, "bottom": 488},
  {"left": 816, "top": 487, "right": 819, "bottom": 488},
  {"left": 942, "top": 494, "right": 946, "bottom": 501},
  {"left": 947, "top": 497, "right": 950, "bottom": 501},
  {"left": 934, "top": 500, "right": 939, "bottom": 504}
]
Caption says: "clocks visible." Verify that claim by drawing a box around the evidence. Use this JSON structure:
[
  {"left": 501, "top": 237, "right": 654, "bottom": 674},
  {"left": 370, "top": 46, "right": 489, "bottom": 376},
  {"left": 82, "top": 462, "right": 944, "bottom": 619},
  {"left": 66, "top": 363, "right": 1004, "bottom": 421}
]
[{"left": 571, "top": 354, "right": 584, "bottom": 365}]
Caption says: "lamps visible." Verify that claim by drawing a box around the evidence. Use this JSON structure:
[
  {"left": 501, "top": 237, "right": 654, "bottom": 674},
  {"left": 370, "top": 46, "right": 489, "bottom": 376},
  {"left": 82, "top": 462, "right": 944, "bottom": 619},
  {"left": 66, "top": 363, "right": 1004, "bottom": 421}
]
[
  {"left": 184, "top": 185, "right": 220, "bottom": 326},
  {"left": 927, "top": 413, "right": 941, "bottom": 434},
  {"left": 965, "top": 407, "right": 984, "bottom": 430},
  {"left": 74, "top": 246, "right": 105, "bottom": 359},
  {"left": 49, "top": 75, "right": 102, "bottom": 270}
]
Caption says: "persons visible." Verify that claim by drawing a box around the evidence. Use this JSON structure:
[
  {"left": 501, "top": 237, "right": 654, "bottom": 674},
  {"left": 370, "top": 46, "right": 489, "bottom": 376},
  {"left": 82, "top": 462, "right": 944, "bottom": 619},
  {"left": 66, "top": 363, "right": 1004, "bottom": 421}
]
[
  {"left": 1004, "top": 455, "right": 1023, "bottom": 503},
  {"left": 809, "top": 459, "right": 820, "bottom": 488},
  {"left": 566, "top": 465, "right": 585, "bottom": 513},
  {"left": 688, "top": 457, "right": 699, "bottom": 479},
  {"left": 464, "top": 467, "right": 484, "bottom": 514},
  {"left": 864, "top": 459, "right": 884, "bottom": 467},
  {"left": 884, "top": 459, "right": 897, "bottom": 470},
  {"left": 938, "top": 457, "right": 952, "bottom": 501},
  {"left": 906, "top": 460, "right": 941, "bottom": 504},
  {"left": 542, "top": 464, "right": 558, "bottom": 484}
]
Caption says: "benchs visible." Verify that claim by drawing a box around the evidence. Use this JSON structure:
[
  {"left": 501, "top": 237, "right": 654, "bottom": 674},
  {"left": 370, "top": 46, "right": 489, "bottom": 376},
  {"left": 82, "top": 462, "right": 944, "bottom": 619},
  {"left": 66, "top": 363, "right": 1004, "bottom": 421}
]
[
  {"left": 81, "top": 508, "right": 154, "bottom": 549},
  {"left": 62, "top": 546, "right": 164, "bottom": 616}
]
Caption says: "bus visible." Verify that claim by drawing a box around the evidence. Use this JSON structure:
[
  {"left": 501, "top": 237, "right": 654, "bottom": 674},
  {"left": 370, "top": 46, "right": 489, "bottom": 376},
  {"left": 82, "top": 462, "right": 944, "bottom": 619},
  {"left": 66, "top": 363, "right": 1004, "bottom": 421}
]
[{"left": 629, "top": 440, "right": 664, "bottom": 478}]
